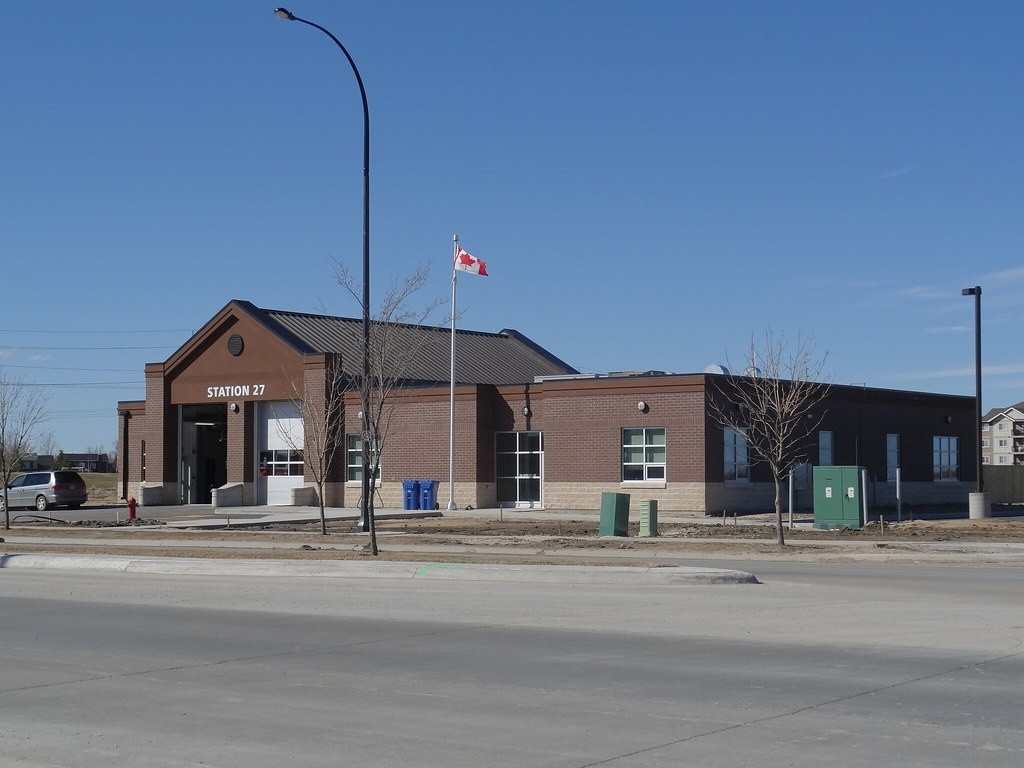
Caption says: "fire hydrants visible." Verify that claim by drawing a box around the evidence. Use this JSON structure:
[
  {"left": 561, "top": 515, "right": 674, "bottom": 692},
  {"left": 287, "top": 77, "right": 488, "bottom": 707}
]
[{"left": 128, "top": 497, "right": 139, "bottom": 521}]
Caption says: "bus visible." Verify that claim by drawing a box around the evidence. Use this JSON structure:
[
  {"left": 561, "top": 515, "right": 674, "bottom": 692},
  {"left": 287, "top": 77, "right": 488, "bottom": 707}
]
[{"left": 61, "top": 467, "right": 83, "bottom": 473}]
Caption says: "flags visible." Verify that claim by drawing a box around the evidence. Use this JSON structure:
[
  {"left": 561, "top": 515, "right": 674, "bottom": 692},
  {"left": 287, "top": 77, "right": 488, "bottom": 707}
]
[{"left": 453, "top": 249, "right": 488, "bottom": 276}]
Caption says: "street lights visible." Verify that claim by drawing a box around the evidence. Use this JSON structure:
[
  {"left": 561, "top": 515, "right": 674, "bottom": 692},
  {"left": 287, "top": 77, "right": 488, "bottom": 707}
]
[
  {"left": 960, "top": 284, "right": 994, "bottom": 520},
  {"left": 274, "top": 4, "right": 374, "bottom": 532}
]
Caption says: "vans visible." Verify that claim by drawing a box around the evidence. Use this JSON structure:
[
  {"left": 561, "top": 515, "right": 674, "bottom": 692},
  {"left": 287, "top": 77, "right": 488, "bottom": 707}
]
[{"left": 0, "top": 471, "right": 88, "bottom": 511}]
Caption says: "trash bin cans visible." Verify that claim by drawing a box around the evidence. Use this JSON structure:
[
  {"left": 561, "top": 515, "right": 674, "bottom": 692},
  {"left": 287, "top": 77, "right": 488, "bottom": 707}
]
[
  {"left": 419, "top": 480, "right": 440, "bottom": 510},
  {"left": 403, "top": 479, "right": 419, "bottom": 509}
]
